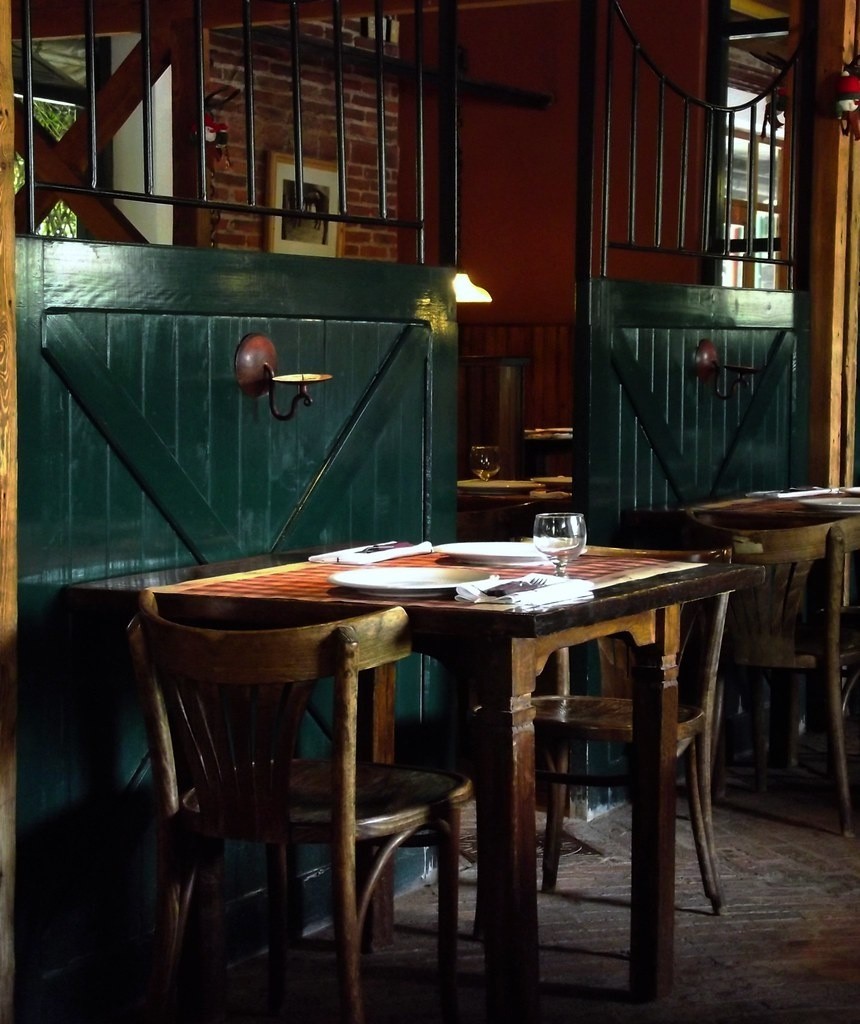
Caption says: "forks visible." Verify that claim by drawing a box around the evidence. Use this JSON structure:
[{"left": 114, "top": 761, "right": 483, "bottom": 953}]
[{"left": 487, "top": 577, "right": 548, "bottom": 598}]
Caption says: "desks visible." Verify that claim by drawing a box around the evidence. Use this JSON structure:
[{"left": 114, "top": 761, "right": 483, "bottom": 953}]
[
  {"left": 62, "top": 541, "right": 766, "bottom": 1024},
  {"left": 454, "top": 486, "right": 572, "bottom": 516},
  {"left": 699, "top": 491, "right": 856, "bottom": 768}
]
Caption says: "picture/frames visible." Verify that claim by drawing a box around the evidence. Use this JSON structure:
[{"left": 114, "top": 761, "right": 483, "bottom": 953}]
[{"left": 266, "top": 151, "right": 346, "bottom": 258}]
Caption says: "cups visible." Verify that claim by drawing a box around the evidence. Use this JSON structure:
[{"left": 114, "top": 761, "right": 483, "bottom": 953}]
[{"left": 468, "top": 445, "right": 503, "bottom": 482}]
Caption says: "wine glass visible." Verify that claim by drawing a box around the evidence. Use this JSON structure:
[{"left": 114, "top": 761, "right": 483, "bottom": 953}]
[{"left": 532, "top": 512, "right": 588, "bottom": 579}]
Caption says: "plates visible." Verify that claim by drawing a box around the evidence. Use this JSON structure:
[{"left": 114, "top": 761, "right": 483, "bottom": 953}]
[
  {"left": 432, "top": 540, "right": 589, "bottom": 570},
  {"left": 327, "top": 565, "right": 501, "bottom": 598},
  {"left": 797, "top": 497, "right": 860, "bottom": 510},
  {"left": 530, "top": 476, "right": 572, "bottom": 483},
  {"left": 456, "top": 479, "right": 546, "bottom": 495},
  {"left": 839, "top": 486, "right": 860, "bottom": 494}
]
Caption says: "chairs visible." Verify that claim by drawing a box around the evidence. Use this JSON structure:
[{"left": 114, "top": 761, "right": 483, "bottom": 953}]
[
  {"left": 526, "top": 508, "right": 736, "bottom": 919},
  {"left": 683, "top": 509, "right": 859, "bottom": 840},
  {"left": 123, "top": 580, "right": 472, "bottom": 1023}
]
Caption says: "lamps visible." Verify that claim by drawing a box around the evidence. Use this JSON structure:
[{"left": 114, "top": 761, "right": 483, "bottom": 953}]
[
  {"left": 698, "top": 340, "right": 756, "bottom": 400},
  {"left": 235, "top": 333, "right": 334, "bottom": 421}
]
